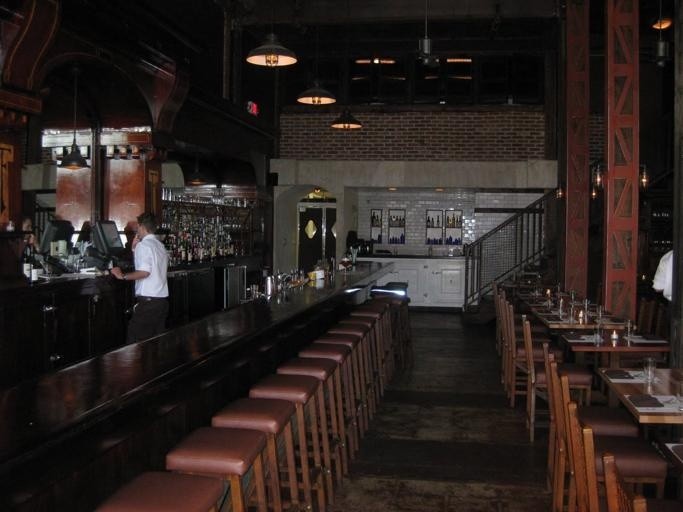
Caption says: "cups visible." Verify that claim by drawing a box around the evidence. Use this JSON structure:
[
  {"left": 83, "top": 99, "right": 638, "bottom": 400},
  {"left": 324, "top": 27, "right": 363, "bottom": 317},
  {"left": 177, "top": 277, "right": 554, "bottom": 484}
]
[
  {"left": 532, "top": 284, "right": 683, "bottom": 411},
  {"left": 250, "top": 284, "right": 258, "bottom": 300}
]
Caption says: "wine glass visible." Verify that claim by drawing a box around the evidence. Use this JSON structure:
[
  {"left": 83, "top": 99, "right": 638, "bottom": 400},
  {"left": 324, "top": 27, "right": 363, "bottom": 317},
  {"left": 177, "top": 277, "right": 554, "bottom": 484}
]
[
  {"left": 341, "top": 256, "right": 351, "bottom": 276},
  {"left": 324, "top": 259, "right": 330, "bottom": 280}
]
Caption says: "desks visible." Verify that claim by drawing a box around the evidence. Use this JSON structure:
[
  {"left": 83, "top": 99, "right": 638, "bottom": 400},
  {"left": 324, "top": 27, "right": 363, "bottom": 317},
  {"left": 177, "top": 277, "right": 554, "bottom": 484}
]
[
  {"left": 513, "top": 286, "right": 671, "bottom": 410},
  {"left": 596, "top": 362, "right": 683, "bottom": 512}
]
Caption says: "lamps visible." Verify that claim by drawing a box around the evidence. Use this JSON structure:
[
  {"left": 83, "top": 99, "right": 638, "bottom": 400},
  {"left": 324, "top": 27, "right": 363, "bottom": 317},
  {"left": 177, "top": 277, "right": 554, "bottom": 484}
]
[
  {"left": 331, "top": 108, "right": 363, "bottom": 130},
  {"left": 555, "top": 185, "right": 564, "bottom": 199},
  {"left": 417, "top": 0, "right": 433, "bottom": 67},
  {"left": 650, "top": 14, "right": 672, "bottom": 32},
  {"left": 59, "top": 70, "right": 87, "bottom": 170},
  {"left": 590, "top": 163, "right": 650, "bottom": 202},
  {"left": 245, "top": 1, "right": 298, "bottom": 68},
  {"left": 296, "top": 79, "right": 337, "bottom": 106},
  {"left": 654, "top": 1, "right": 669, "bottom": 57}
]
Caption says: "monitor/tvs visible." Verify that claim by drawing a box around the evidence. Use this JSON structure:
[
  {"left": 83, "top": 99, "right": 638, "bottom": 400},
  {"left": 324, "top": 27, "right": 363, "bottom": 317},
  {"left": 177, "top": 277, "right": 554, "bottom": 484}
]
[
  {"left": 93, "top": 219, "right": 123, "bottom": 259},
  {"left": 39, "top": 219, "right": 75, "bottom": 253}
]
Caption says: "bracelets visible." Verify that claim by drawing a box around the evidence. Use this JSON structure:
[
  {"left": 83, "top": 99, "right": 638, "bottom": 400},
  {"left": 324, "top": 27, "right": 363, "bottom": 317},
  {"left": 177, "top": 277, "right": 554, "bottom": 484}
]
[{"left": 121, "top": 272, "right": 127, "bottom": 281}]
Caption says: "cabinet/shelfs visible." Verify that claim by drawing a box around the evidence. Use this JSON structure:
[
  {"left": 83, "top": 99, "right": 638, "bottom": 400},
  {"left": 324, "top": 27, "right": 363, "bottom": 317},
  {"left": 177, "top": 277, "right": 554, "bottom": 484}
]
[
  {"left": 159, "top": 200, "right": 253, "bottom": 267},
  {"left": 370, "top": 209, "right": 406, "bottom": 244},
  {"left": 340, "top": 257, "right": 479, "bottom": 313},
  {"left": 425, "top": 209, "right": 463, "bottom": 245}
]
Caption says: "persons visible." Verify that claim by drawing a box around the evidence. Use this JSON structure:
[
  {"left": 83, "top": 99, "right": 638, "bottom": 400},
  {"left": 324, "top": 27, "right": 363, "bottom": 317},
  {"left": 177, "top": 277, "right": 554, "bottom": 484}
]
[
  {"left": 19, "top": 217, "right": 46, "bottom": 275},
  {"left": 652, "top": 248, "right": 674, "bottom": 347},
  {"left": 110, "top": 212, "right": 170, "bottom": 345}
]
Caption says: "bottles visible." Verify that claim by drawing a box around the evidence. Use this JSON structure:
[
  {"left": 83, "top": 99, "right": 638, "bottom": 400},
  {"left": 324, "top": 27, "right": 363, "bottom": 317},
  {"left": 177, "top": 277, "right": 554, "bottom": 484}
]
[
  {"left": 161, "top": 188, "right": 249, "bottom": 266},
  {"left": 429, "top": 247, "right": 432, "bottom": 256},
  {"left": 372, "top": 211, "right": 461, "bottom": 244},
  {"left": 275, "top": 268, "right": 304, "bottom": 293},
  {"left": 394, "top": 248, "right": 397, "bottom": 256}
]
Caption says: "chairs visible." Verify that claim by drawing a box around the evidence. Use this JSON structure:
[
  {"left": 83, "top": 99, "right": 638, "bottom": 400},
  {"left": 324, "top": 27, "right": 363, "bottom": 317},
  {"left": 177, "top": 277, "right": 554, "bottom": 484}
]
[
  {"left": 543, "top": 341, "right": 683, "bottom": 512},
  {"left": 492, "top": 278, "right": 596, "bottom": 441}
]
